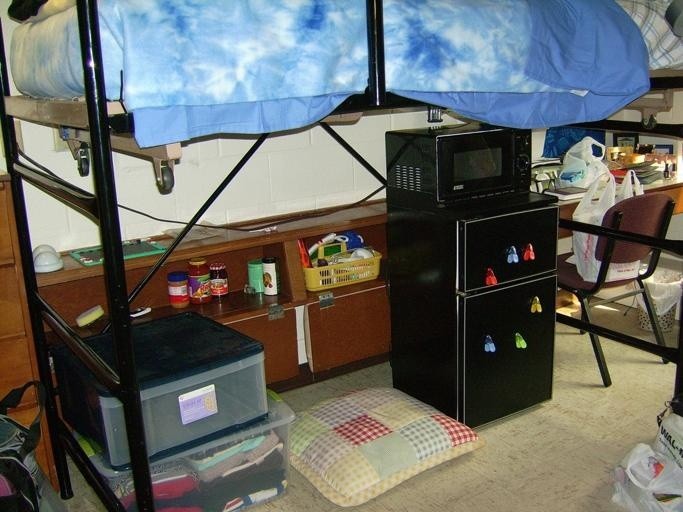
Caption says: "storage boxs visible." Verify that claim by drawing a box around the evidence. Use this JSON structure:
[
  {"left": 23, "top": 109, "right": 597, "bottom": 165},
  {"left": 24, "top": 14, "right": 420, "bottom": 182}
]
[
  {"left": 52, "top": 311, "right": 269, "bottom": 471},
  {"left": 68, "top": 387, "right": 297, "bottom": 509}
]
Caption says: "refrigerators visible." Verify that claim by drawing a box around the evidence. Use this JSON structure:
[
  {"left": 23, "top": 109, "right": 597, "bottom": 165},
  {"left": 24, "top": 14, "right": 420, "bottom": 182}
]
[{"left": 385, "top": 191, "right": 560, "bottom": 430}]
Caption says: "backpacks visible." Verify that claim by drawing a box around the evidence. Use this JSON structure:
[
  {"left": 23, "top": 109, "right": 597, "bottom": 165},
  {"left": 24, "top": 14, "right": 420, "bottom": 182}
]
[{"left": 0, "top": 415, "right": 46, "bottom": 511}]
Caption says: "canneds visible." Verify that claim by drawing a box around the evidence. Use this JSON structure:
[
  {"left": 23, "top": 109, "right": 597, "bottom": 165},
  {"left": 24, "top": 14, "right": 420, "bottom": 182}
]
[
  {"left": 209, "top": 262, "right": 229, "bottom": 300},
  {"left": 187, "top": 257, "right": 212, "bottom": 305}
]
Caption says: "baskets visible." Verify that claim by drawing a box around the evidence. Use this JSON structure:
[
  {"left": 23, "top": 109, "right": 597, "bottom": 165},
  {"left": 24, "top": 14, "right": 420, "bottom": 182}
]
[{"left": 304, "top": 249, "right": 383, "bottom": 291}]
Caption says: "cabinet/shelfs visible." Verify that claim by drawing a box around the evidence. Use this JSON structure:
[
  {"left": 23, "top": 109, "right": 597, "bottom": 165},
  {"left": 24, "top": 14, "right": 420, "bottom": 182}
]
[
  {"left": 1, "top": 170, "right": 60, "bottom": 496},
  {"left": 38, "top": 174, "right": 683, "bottom": 351}
]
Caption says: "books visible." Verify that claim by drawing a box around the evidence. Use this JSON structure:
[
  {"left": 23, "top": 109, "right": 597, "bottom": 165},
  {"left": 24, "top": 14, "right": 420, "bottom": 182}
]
[
  {"left": 544, "top": 186, "right": 590, "bottom": 201},
  {"left": 531, "top": 158, "right": 562, "bottom": 169}
]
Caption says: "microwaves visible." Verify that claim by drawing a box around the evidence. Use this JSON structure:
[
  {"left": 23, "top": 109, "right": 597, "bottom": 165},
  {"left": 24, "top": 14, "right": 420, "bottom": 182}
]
[{"left": 386, "top": 128, "right": 532, "bottom": 208}]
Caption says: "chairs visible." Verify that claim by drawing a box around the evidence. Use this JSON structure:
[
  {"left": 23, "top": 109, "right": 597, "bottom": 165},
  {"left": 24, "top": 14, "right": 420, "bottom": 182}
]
[{"left": 557, "top": 191, "right": 676, "bottom": 389}]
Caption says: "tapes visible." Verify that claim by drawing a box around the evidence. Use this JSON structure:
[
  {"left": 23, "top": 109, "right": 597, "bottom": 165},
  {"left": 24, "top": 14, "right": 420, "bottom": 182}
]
[{"left": 74, "top": 305, "right": 105, "bottom": 328}]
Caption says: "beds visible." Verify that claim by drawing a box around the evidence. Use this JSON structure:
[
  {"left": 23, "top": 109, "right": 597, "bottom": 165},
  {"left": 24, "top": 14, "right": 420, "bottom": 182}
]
[{"left": 0, "top": 0, "right": 683, "bottom": 510}]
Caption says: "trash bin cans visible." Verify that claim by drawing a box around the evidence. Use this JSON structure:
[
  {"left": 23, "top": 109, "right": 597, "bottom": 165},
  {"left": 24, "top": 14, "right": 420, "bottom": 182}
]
[{"left": 636, "top": 267, "right": 683, "bottom": 333}]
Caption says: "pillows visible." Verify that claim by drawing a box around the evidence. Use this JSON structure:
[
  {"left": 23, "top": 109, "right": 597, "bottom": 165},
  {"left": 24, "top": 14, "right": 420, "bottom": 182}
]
[{"left": 281, "top": 384, "right": 487, "bottom": 508}]
